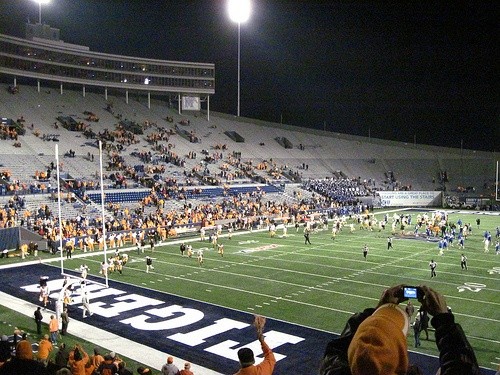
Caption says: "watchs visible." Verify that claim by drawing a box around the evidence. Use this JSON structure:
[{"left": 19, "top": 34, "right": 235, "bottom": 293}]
[{"left": 446, "top": 306, "right": 452, "bottom": 313}]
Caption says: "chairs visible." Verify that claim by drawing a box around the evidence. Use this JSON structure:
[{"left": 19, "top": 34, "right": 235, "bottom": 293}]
[{"left": 0, "top": 86, "right": 500, "bottom": 234}]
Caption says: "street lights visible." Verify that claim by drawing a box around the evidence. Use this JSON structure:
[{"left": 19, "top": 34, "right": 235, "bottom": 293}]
[
  {"left": 228, "top": 0, "right": 251, "bottom": 116},
  {"left": 32, "top": 0, "right": 50, "bottom": 25}
]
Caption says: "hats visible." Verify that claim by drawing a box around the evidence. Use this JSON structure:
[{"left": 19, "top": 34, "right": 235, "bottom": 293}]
[
  {"left": 237, "top": 348, "right": 255, "bottom": 366},
  {"left": 16, "top": 340, "right": 34, "bottom": 359},
  {"left": 0, "top": 328, "right": 194, "bottom": 374},
  {"left": 347, "top": 303, "right": 409, "bottom": 375}
]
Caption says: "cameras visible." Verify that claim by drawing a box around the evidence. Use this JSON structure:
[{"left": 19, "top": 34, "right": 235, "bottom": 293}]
[{"left": 402, "top": 286, "right": 425, "bottom": 299}]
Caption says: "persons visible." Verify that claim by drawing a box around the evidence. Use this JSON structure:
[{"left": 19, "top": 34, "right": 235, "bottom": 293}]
[{"left": 0, "top": 86, "right": 500, "bottom": 375}]
